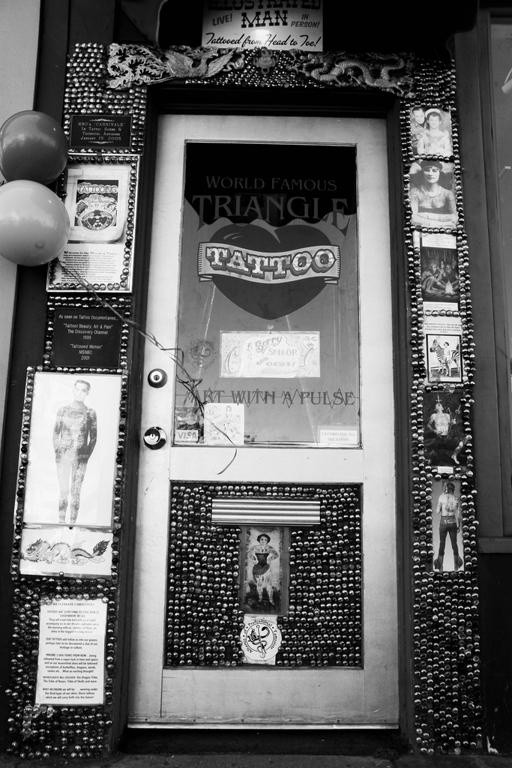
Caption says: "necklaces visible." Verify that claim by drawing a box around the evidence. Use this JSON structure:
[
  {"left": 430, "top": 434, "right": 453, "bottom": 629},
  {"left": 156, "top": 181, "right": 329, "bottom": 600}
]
[{"left": 420, "top": 183, "right": 442, "bottom": 197}]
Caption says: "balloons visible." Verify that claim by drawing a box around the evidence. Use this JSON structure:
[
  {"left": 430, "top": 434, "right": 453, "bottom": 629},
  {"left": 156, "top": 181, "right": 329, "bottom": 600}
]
[
  {"left": 0, "top": 179, "right": 70, "bottom": 266},
  {"left": 1, "top": 110, "right": 67, "bottom": 186}
]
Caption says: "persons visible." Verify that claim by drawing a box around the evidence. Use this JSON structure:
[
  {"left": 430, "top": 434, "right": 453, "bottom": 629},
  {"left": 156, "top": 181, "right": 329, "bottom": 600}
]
[
  {"left": 411, "top": 107, "right": 452, "bottom": 154},
  {"left": 248, "top": 534, "right": 279, "bottom": 606},
  {"left": 52, "top": 379, "right": 97, "bottom": 522},
  {"left": 422, "top": 257, "right": 458, "bottom": 294},
  {"left": 409, "top": 159, "right": 456, "bottom": 215},
  {"left": 426, "top": 402, "right": 463, "bottom": 464},
  {"left": 431, "top": 339, "right": 459, "bottom": 375},
  {"left": 434, "top": 482, "right": 463, "bottom": 572}
]
